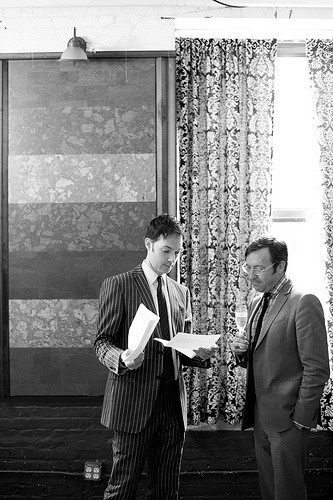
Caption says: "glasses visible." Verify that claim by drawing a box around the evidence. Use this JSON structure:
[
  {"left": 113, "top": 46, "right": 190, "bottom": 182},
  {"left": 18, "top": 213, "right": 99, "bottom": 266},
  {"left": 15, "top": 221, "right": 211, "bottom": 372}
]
[{"left": 242, "top": 261, "right": 277, "bottom": 275}]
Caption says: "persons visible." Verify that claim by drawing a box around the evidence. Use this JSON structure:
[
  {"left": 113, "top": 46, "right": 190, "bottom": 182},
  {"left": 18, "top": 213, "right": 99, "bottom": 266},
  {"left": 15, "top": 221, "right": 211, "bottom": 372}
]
[
  {"left": 94, "top": 215, "right": 219, "bottom": 500},
  {"left": 229, "top": 237, "right": 330, "bottom": 500}
]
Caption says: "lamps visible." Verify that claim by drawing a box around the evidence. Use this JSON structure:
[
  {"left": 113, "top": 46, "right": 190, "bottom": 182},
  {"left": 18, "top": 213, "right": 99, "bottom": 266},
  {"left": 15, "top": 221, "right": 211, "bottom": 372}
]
[{"left": 59, "top": 26, "right": 89, "bottom": 62}]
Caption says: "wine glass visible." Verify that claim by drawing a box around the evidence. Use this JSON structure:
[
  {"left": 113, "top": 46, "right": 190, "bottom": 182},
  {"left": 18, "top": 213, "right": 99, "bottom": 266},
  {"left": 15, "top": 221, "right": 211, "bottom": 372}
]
[{"left": 235, "top": 304, "right": 248, "bottom": 351}]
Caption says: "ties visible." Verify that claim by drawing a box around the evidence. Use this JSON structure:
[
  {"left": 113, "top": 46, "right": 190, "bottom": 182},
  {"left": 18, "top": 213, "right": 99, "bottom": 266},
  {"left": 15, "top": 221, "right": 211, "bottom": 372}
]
[
  {"left": 248, "top": 292, "right": 269, "bottom": 378},
  {"left": 156, "top": 275, "right": 176, "bottom": 389}
]
